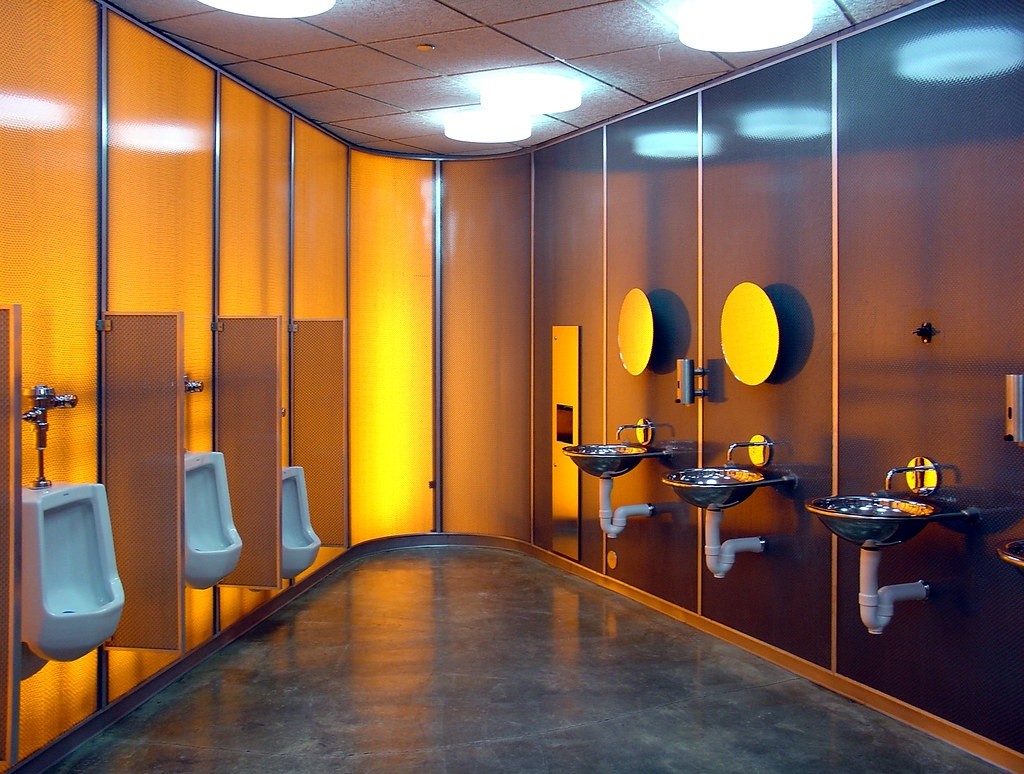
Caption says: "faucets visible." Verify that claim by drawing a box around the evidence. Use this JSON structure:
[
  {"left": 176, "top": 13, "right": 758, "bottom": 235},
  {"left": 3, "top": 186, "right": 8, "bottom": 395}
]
[
  {"left": 726, "top": 441, "right": 760, "bottom": 461},
  {"left": 884, "top": 466, "right": 922, "bottom": 491},
  {"left": 941, "top": 463, "right": 963, "bottom": 484},
  {"left": 614, "top": 423, "right": 636, "bottom": 441},
  {"left": 656, "top": 422, "right": 676, "bottom": 436},
  {"left": 774, "top": 439, "right": 794, "bottom": 455}
]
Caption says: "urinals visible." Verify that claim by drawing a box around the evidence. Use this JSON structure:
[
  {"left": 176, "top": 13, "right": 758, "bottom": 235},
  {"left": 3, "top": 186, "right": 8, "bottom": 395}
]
[
  {"left": 19, "top": 480, "right": 126, "bottom": 664},
  {"left": 281, "top": 466, "right": 321, "bottom": 578},
  {"left": 184, "top": 449, "right": 242, "bottom": 590}
]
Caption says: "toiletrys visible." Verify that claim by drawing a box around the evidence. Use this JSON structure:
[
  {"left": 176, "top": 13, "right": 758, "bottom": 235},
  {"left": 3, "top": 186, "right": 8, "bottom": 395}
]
[
  {"left": 1002, "top": 371, "right": 1023, "bottom": 443},
  {"left": 675, "top": 358, "right": 696, "bottom": 407}
]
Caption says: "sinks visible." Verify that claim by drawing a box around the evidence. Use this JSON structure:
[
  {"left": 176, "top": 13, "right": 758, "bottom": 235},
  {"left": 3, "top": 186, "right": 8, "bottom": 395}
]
[
  {"left": 802, "top": 493, "right": 941, "bottom": 551},
  {"left": 562, "top": 443, "right": 647, "bottom": 478},
  {"left": 998, "top": 537, "right": 1023, "bottom": 573},
  {"left": 661, "top": 467, "right": 765, "bottom": 509}
]
